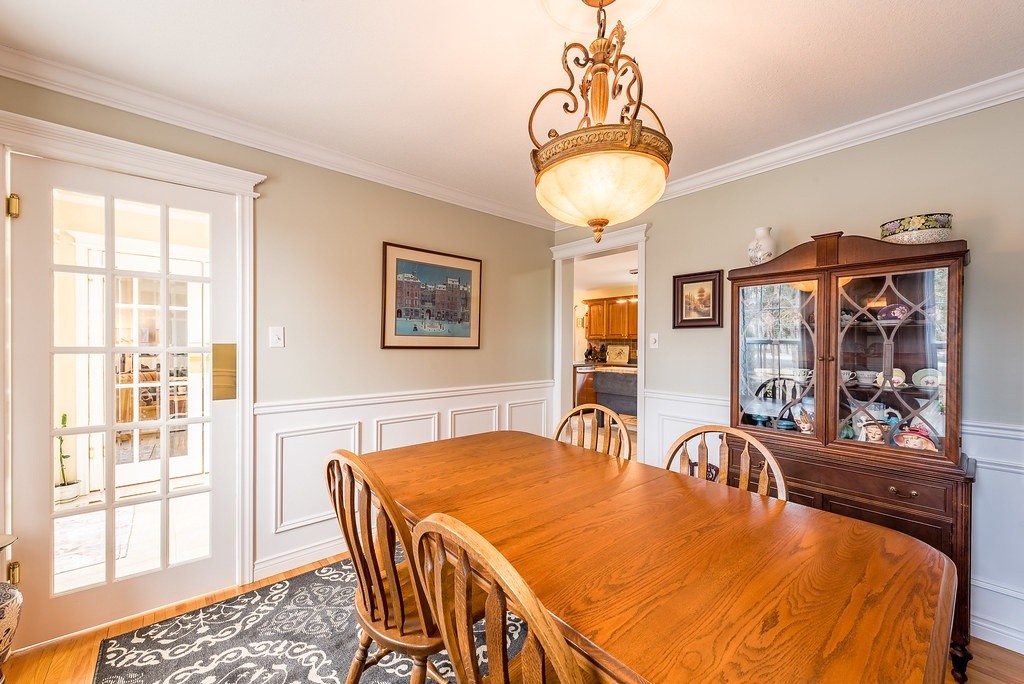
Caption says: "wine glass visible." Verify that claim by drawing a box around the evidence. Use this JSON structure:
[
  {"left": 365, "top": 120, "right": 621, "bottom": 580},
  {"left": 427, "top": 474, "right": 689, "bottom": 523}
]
[{"left": 752, "top": 414, "right": 769, "bottom": 427}]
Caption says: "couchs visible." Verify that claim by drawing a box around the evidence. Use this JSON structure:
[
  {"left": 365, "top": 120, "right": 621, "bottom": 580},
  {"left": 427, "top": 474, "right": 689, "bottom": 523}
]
[{"left": 117, "top": 373, "right": 162, "bottom": 439}]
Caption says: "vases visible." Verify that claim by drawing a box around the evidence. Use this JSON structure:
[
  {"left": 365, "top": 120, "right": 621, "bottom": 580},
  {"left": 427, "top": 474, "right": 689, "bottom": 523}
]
[
  {"left": 879, "top": 212, "right": 953, "bottom": 245},
  {"left": 747, "top": 226, "right": 778, "bottom": 266},
  {"left": 0, "top": 534, "right": 24, "bottom": 666}
]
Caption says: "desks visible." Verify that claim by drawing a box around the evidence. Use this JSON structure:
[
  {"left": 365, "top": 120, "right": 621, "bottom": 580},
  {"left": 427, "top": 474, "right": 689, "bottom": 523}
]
[{"left": 358, "top": 430, "right": 958, "bottom": 684}]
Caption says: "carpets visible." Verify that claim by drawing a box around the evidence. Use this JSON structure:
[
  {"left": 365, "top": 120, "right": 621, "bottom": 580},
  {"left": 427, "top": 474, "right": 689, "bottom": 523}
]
[{"left": 93, "top": 540, "right": 528, "bottom": 684}]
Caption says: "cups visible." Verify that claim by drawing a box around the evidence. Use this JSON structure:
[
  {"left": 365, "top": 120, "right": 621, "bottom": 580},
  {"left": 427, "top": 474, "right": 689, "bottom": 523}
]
[
  {"left": 840, "top": 370, "right": 856, "bottom": 383},
  {"left": 856, "top": 371, "right": 878, "bottom": 384},
  {"left": 802, "top": 398, "right": 814, "bottom": 415},
  {"left": 754, "top": 368, "right": 814, "bottom": 378},
  {"left": 915, "top": 306, "right": 925, "bottom": 319}
]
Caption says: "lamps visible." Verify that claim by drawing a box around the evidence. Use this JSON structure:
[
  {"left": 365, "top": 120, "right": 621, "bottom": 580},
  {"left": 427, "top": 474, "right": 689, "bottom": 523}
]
[{"left": 528, "top": 0, "right": 673, "bottom": 243}]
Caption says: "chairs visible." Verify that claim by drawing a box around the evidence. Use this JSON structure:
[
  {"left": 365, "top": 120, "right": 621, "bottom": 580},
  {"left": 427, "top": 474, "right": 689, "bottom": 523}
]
[
  {"left": 554, "top": 403, "right": 631, "bottom": 460},
  {"left": 593, "top": 367, "right": 637, "bottom": 427},
  {"left": 665, "top": 425, "right": 789, "bottom": 502},
  {"left": 323, "top": 449, "right": 585, "bottom": 684}
]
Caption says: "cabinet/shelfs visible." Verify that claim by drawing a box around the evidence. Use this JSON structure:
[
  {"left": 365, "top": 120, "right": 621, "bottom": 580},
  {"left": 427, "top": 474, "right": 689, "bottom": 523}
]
[
  {"left": 581, "top": 295, "right": 638, "bottom": 340},
  {"left": 719, "top": 231, "right": 976, "bottom": 684}
]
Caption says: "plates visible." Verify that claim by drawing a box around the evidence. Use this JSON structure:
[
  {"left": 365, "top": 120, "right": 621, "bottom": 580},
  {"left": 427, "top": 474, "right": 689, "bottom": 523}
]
[
  {"left": 857, "top": 383, "right": 877, "bottom": 388},
  {"left": 750, "top": 377, "right": 809, "bottom": 382},
  {"left": 915, "top": 386, "right": 943, "bottom": 391},
  {"left": 873, "top": 383, "right": 912, "bottom": 391},
  {"left": 841, "top": 318, "right": 877, "bottom": 325},
  {"left": 877, "top": 304, "right": 909, "bottom": 321}
]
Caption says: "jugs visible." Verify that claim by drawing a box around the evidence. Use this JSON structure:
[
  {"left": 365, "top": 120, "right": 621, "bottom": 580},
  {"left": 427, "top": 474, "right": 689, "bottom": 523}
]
[{"left": 847, "top": 399, "right": 902, "bottom": 439}]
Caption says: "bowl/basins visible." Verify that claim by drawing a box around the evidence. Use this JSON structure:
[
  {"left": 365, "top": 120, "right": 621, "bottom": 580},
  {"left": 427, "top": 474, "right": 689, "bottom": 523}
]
[
  {"left": 912, "top": 368, "right": 942, "bottom": 386},
  {"left": 769, "top": 420, "right": 794, "bottom": 430},
  {"left": 880, "top": 213, "right": 953, "bottom": 245},
  {"left": 877, "top": 368, "right": 908, "bottom": 388}
]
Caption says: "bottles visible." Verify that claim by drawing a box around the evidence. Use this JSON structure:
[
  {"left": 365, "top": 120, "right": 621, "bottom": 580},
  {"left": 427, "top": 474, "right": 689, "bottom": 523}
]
[
  {"left": 910, "top": 397, "right": 945, "bottom": 437},
  {"left": 841, "top": 305, "right": 874, "bottom": 322},
  {"left": 748, "top": 227, "right": 776, "bottom": 266}
]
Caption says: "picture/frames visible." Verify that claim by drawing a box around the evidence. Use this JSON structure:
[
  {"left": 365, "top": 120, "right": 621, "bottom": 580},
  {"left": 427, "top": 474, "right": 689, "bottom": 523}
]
[
  {"left": 672, "top": 269, "right": 724, "bottom": 329},
  {"left": 381, "top": 241, "right": 483, "bottom": 349}
]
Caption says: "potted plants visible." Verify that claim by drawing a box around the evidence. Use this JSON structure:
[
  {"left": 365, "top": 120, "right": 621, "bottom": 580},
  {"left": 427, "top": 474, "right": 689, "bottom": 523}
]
[{"left": 54, "top": 414, "right": 82, "bottom": 511}]
